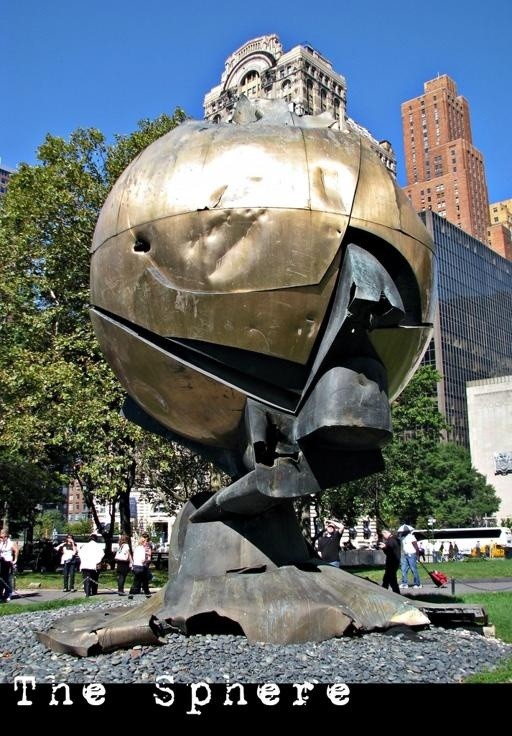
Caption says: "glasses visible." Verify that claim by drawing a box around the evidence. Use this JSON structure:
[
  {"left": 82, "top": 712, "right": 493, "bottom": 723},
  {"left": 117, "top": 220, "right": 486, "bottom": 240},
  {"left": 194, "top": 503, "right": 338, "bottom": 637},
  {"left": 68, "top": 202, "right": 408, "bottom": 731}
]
[{"left": 0, "top": 536, "right": 9, "bottom": 538}]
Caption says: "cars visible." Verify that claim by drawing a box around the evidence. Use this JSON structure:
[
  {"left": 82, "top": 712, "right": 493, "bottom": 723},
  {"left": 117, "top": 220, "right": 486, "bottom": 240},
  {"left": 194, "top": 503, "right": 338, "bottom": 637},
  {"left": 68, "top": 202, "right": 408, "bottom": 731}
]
[
  {"left": 469, "top": 542, "right": 504, "bottom": 558},
  {"left": 16, "top": 540, "right": 84, "bottom": 574}
]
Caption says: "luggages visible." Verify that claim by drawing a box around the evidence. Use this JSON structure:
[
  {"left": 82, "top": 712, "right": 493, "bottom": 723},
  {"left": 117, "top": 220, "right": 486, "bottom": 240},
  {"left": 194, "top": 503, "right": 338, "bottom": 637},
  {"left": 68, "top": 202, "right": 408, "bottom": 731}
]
[{"left": 419, "top": 561, "right": 448, "bottom": 588}]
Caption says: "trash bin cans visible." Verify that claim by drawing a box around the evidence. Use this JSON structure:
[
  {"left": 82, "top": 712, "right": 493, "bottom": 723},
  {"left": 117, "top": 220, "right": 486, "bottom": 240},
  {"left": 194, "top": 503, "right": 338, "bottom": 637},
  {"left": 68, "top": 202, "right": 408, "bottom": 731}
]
[{"left": 504, "top": 547, "right": 512, "bottom": 559}]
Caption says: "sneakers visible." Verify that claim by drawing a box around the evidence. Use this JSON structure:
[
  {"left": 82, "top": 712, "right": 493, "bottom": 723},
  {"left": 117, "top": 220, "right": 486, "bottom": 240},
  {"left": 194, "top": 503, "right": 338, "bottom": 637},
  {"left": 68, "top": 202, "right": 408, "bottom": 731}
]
[
  {"left": 146, "top": 596, "right": 152, "bottom": 598},
  {"left": 71, "top": 589, "right": 74, "bottom": 592},
  {"left": 412, "top": 584, "right": 422, "bottom": 588},
  {"left": 63, "top": 589, "right": 67, "bottom": 592},
  {"left": 399, "top": 583, "right": 408, "bottom": 588},
  {"left": 119, "top": 593, "right": 126, "bottom": 596},
  {"left": 128, "top": 596, "right": 133, "bottom": 599},
  {"left": 4, "top": 598, "right": 10, "bottom": 603}
]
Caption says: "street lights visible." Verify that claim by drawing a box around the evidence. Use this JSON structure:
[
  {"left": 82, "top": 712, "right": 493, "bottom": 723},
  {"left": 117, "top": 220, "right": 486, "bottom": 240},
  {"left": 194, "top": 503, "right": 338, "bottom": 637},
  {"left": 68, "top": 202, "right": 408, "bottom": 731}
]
[{"left": 426, "top": 514, "right": 437, "bottom": 563}]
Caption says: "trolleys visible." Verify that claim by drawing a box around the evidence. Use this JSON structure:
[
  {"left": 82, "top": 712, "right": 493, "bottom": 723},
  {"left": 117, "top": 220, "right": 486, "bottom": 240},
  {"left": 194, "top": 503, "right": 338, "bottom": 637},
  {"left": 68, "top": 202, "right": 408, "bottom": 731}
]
[{"left": 412, "top": 555, "right": 447, "bottom": 590}]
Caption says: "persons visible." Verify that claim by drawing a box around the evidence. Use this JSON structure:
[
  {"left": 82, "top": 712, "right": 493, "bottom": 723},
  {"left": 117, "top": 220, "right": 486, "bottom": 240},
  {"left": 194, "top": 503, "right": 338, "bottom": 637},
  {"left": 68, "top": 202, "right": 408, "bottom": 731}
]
[
  {"left": 60, "top": 535, "right": 77, "bottom": 592},
  {"left": 476, "top": 539, "right": 496, "bottom": 560},
  {"left": 0, "top": 529, "right": 20, "bottom": 603},
  {"left": 317, "top": 520, "right": 345, "bottom": 568},
  {"left": 378, "top": 529, "right": 401, "bottom": 594},
  {"left": 78, "top": 534, "right": 106, "bottom": 596},
  {"left": 417, "top": 542, "right": 425, "bottom": 562},
  {"left": 398, "top": 531, "right": 422, "bottom": 588},
  {"left": 114, "top": 533, "right": 154, "bottom": 599},
  {"left": 439, "top": 541, "right": 459, "bottom": 562}
]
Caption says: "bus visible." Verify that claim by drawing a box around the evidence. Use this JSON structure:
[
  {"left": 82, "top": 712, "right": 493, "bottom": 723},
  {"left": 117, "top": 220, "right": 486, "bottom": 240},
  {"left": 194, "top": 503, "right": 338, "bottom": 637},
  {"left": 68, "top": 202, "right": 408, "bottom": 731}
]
[
  {"left": 52, "top": 533, "right": 120, "bottom": 571},
  {"left": 409, "top": 526, "right": 511, "bottom": 566}
]
